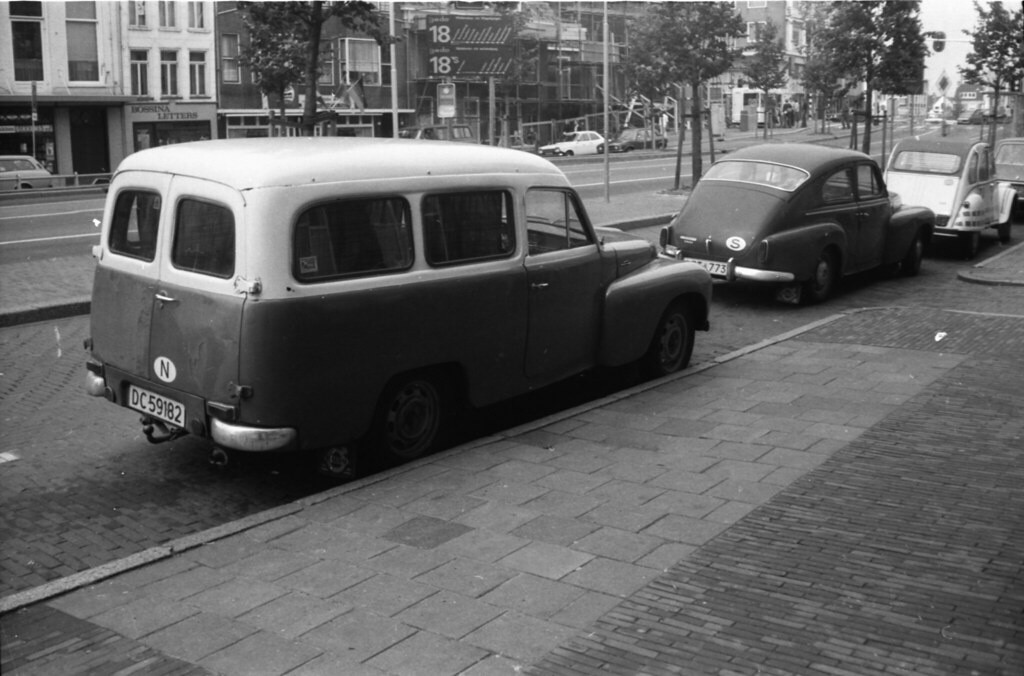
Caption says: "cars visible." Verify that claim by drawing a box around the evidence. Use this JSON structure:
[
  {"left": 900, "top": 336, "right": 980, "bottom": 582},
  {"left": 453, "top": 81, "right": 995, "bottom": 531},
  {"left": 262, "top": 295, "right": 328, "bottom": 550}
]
[
  {"left": 992, "top": 138, "right": 1024, "bottom": 225},
  {"left": 539, "top": 131, "right": 606, "bottom": 156},
  {"left": 598, "top": 129, "right": 667, "bottom": 155},
  {"left": 881, "top": 138, "right": 1018, "bottom": 260},
  {"left": 659, "top": 143, "right": 934, "bottom": 302}
]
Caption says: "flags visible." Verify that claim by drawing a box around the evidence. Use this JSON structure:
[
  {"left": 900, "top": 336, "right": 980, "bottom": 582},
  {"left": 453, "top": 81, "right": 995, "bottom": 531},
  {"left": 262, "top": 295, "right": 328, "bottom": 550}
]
[
  {"left": 335, "top": 80, "right": 350, "bottom": 105},
  {"left": 348, "top": 78, "right": 367, "bottom": 112}
]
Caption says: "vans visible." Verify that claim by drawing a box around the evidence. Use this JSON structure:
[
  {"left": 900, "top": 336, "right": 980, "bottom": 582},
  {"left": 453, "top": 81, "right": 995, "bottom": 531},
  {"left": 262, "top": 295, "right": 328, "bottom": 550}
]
[
  {"left": 0, "top": 155, "right": 52, "bottom": 191},
  {"left": 399, "top": 125, "right": 476, "bottom": 142},
  {"left": 958, "top": 109, "right": 983, "bottom": 124},
  {"left": 84, "top": 136, "right": 713, "bottom": 466}
]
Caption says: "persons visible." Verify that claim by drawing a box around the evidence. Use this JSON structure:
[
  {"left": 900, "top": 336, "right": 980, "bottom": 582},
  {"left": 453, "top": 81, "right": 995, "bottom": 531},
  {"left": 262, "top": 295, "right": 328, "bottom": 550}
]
[
  {"left": 783, "top": 99, "right": 799, "bottom": 128},
  {"left": 841, "top": 102, "right": 851, "bottom": 129}
]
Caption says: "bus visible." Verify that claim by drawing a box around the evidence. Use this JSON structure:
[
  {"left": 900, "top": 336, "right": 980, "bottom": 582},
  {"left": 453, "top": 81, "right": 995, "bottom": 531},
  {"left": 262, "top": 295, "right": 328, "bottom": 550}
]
[{"left": 731, "top": 87, "right": 867, "bottom": 128}]
